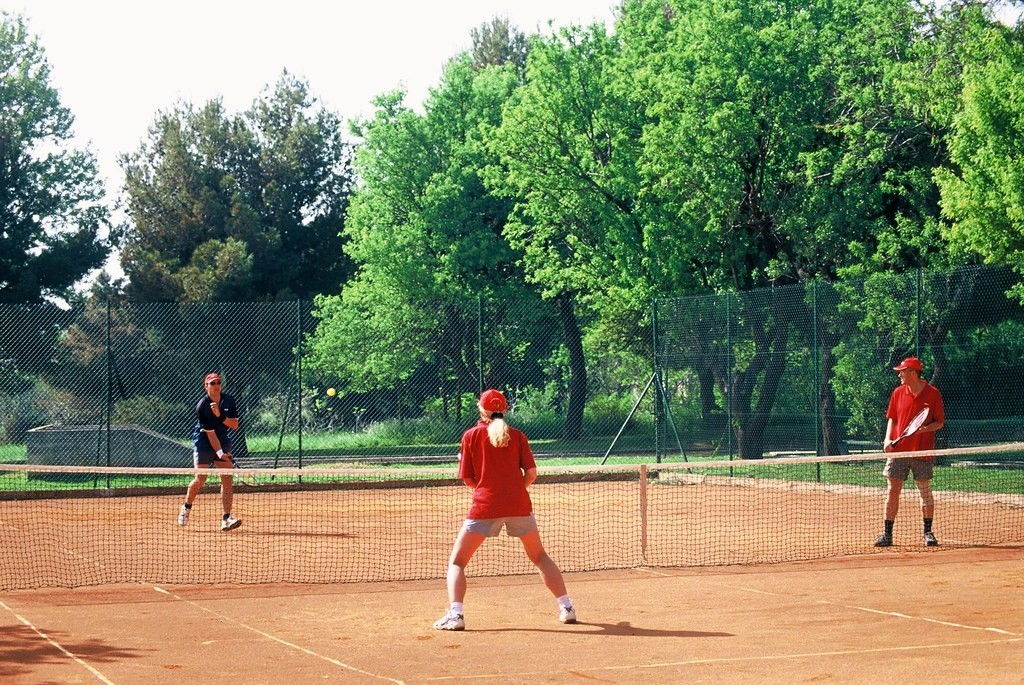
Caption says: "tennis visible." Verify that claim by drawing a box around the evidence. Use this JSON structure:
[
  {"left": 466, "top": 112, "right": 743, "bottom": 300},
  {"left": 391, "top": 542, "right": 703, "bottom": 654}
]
[{"left": 326, "top": 387, "right": 336, "bottom": 397}]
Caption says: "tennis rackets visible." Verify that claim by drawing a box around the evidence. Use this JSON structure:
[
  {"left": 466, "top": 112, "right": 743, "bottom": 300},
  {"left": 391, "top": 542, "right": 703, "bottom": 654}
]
[
  {"left": 892, "top": 406, "right": 930, "bottom": 447},
  {"left": 228, "top": 455, "right": 260, "bottom": 487}
]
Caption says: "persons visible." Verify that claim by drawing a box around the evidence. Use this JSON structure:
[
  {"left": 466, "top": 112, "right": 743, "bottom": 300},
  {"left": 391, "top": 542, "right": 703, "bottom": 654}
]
[
  {"left": 178, "top": 373, "right": 242, "bottom": 531},
  {"left": 872, "top": 356, "right": 945, "bottom": 545},
  {"left": 433, "top": 389, "right": 577, "bottom": 630}
]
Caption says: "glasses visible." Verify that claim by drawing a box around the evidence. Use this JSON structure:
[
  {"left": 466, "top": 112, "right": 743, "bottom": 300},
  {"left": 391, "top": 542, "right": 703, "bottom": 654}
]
[{"left": 207, "top": 381, "right": 221, "bottom": 385}]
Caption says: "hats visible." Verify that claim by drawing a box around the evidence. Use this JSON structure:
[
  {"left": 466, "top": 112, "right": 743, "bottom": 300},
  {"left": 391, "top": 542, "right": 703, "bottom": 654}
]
[
  {"left": 205, "top": 373, "right": 221, "bottom": 383},
  {"left": 893, "top": 357, "right": 922, "bottom": 371},
  {"left": 477, "top": 389, "right": 508, "bottom": 411}
]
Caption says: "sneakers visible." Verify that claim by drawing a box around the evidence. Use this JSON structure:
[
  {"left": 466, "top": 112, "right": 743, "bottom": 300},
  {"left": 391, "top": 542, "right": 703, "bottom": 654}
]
[
  {"left": 560, "top": 607, "right": 576, "bottom": 623},
  {"left": 924, "top": 532, "right": 937, "bottom": 546},
  {"left": 222, "top": 515, "right": 242, "bottom": 531},
  {"left": 179, "top": 504, "right": 191, "bottom": 526},
  {"left": 875, "top": 534, "right": 893, "bottom": 547},
  {"left": 433, "top": 612, "right": 465, "bottom": 631}
]
